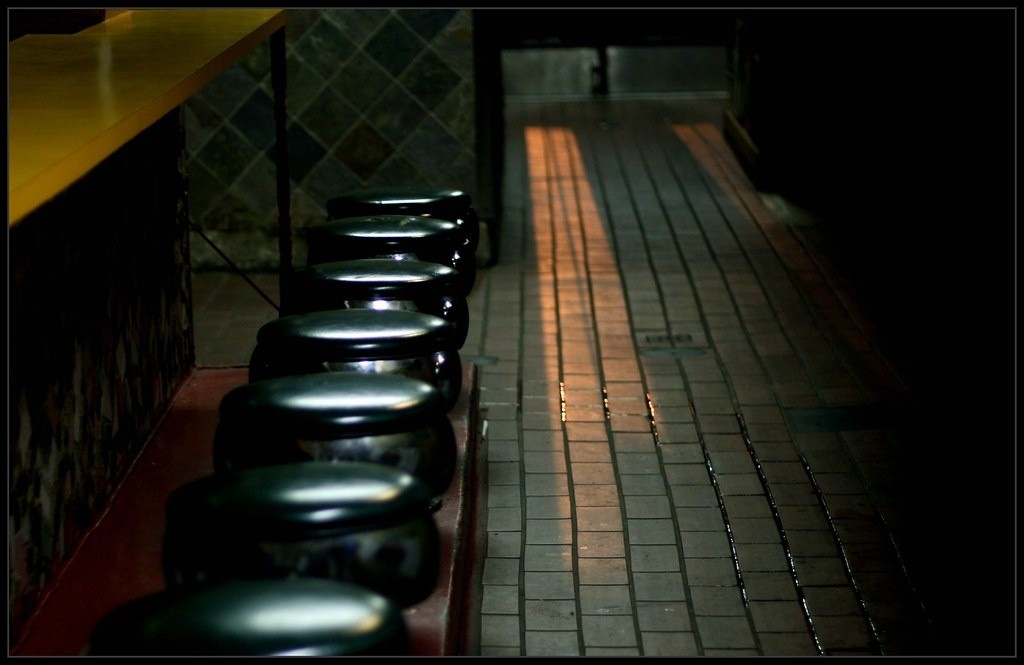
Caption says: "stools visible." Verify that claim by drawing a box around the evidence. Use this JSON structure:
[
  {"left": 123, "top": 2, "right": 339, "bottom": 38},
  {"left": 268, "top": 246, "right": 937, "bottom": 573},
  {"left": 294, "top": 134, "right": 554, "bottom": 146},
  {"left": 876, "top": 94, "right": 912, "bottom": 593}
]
[
  {"left": 299, "top": 215, "right": 463, "bottom": 271},
  {"left": 249, "top": 309, "right": 460, "bottom": 412},
  {"left": 327, "top": 190, "right": 482, "bottom": 254},
  {"left": 211, "top": 370, "right": 460, "bottom": 498},
  {"left": 278, "top": 258, "right": 469, "bottom": 350},
  {"left": 91, "top": 578, "right": 420, "bottom": 656},
  {"left": 160, "top": 462, "right": 444, "bottom": 612}
]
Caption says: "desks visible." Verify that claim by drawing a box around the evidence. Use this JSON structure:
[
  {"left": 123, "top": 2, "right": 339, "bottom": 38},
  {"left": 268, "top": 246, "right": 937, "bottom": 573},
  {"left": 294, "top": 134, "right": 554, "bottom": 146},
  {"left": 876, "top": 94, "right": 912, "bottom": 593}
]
[{"left": 8, "top": 8, "right": 296, "bottom": 318}]
